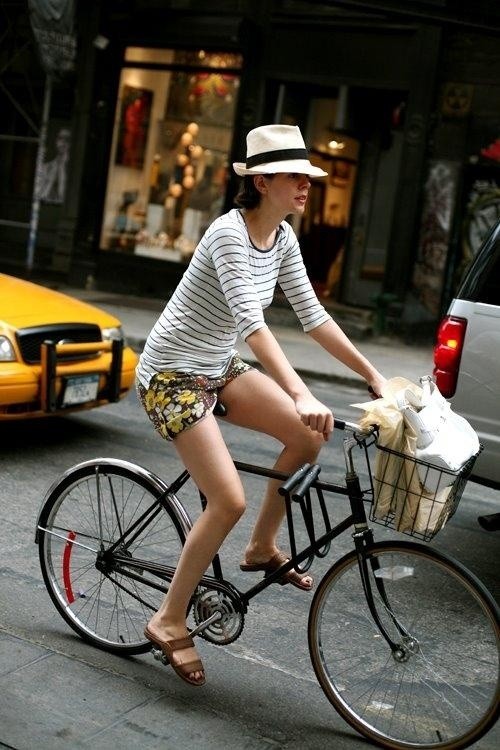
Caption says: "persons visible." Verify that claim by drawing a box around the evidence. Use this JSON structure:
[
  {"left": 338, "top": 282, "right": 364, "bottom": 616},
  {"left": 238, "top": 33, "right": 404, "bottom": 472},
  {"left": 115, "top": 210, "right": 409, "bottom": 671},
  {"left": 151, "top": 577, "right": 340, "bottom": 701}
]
[
  {"left": 134, "top": 124, "right": 386, "bottom": 686},
  {"left": 119, "top": 94, "right": 148, "bottom": 166}
]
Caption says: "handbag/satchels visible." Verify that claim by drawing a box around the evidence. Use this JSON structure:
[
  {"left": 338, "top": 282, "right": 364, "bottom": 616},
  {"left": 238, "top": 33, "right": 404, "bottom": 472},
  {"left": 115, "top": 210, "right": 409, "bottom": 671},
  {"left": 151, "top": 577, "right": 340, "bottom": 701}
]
[
  {"left": 396, "top": 373, "right": 482, "bottom": 495},
  {"left": 349, "top": 375, "right": 458, "bottom": 533}
]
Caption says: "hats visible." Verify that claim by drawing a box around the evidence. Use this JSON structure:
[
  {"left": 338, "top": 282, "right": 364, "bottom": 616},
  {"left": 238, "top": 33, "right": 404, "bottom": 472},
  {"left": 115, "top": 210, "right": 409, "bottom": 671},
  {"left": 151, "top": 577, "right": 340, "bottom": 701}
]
[{"left": 232, "top": 122, "right": 329, "bottom": 180}]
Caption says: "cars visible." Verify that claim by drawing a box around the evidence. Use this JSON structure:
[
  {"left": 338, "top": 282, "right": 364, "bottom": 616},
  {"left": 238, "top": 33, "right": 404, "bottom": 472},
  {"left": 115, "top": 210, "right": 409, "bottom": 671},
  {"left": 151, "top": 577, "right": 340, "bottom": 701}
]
[{"left": 1, "top": 271, "right": 140, "bottom": 423}]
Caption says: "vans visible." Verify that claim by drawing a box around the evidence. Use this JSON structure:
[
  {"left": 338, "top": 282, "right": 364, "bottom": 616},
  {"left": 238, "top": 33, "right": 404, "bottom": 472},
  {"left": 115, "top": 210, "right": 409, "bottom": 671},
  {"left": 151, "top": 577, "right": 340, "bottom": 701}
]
[{"left": 431, "top": 219, "right": 500, "bottom": 542}]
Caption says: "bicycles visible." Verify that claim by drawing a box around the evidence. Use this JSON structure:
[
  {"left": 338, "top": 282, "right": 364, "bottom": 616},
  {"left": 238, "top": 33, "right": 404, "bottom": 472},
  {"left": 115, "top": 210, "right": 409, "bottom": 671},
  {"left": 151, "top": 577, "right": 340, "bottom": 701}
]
[{"left": 29, "top": 332, "right": 497, "bottom": 746}]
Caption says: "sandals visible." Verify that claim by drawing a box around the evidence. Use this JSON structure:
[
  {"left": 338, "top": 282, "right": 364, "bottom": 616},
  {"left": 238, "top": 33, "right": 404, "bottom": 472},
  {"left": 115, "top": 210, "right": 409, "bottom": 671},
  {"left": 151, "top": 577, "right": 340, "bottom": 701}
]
[
  {"left": 143, "top": 624, "right": 207, "bottom": 687},
  {"left": 239, "top": 550, "right": 315, "bottom": 592}
]
